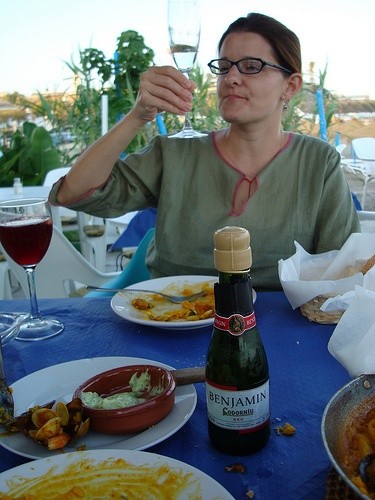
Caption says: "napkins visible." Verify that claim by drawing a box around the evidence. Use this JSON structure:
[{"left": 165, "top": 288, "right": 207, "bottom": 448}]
[{"left": 320, "top": 285, "right": 375, "bottom": 377}]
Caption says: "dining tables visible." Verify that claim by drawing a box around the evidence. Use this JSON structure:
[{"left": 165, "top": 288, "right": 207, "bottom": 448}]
[{"left": 0, "top": 292, "right": 375, "bottom": 500}]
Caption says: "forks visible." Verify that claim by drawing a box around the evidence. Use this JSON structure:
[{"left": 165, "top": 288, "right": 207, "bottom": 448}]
[{"left": 87, "top": 286, "right": 209, "bottom": 305}]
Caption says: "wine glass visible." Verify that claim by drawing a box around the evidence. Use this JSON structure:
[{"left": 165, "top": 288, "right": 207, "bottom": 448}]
[
  {"left": 166, "top": 0, "right": 209, "bottom": 139},
  {"left": 0, "top": 198, "right": 65, "bottom": 342}
]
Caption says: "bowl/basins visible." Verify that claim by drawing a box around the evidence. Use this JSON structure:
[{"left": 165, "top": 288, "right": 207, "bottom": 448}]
[
  {"left": 0, "top": 311, "right": 29, "bottom": 346},
  {"left": 74, "top": 364, "right": 176, "bottom": 435}
]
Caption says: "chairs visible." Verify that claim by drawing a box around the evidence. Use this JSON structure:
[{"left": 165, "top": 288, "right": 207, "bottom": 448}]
[
  {"left": 0, "top": 168, "right": 155, "bottom": 297},
  {"left": 335, "top": 136, "right": 375, "bottom": 212}
]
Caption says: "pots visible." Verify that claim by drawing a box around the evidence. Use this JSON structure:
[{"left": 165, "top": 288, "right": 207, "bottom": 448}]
[{"left": 321, "top": 374, "right": 375, "bottom": 500}]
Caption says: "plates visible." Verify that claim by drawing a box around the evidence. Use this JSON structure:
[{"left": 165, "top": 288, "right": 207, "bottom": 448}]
[
  {"left": 0, "top": 357, "right": 197, "bottom": 459},
  {"left": 0, "top": 450, "right": 235, "bottom": 500},
  {"left": 111, "top": 275, "right": 257, "bottom": 330}
]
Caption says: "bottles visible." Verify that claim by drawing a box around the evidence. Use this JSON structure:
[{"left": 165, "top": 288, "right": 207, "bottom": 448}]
[
  {"left": 205, "top": 226, "right": 269, "bottom": 457},
  {"left": 13, "top": 177, "right": 25, "bottom": 196}
]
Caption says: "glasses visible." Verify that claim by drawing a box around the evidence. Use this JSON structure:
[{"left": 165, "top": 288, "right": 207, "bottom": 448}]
[{"left": 207, "top": 56, "right": 291, "bottom": 75}]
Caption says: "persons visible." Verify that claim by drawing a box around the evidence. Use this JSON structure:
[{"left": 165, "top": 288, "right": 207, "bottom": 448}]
[{"left": 48, "top": 13, "right": 363, "bottom": 293}]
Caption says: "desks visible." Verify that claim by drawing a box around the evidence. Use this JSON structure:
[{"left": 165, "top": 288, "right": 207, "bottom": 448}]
[{"left": 1, "top": 186, "right": 61, "bottom": 234}]
